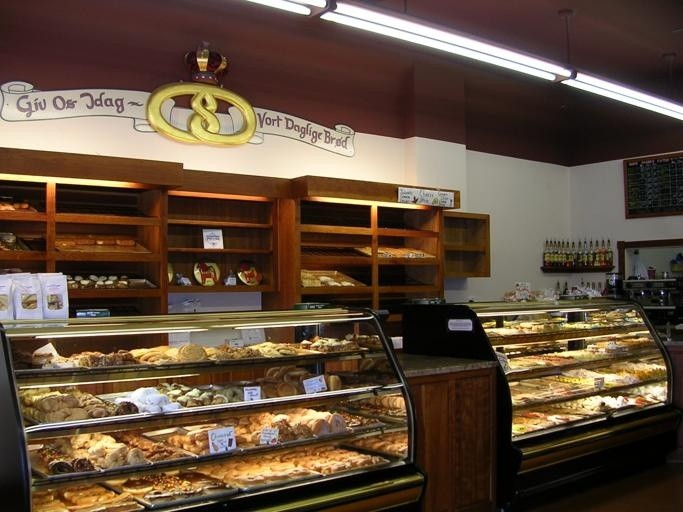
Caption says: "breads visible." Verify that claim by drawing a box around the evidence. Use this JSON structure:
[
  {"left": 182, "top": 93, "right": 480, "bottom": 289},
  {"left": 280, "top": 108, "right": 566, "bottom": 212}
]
[
  {"left": 1, "top": 201, "right": 29, "bottom": 211},
  {"left": 54, "top": 238, "right": 136, "bottom": 247},
  {"left": 480, "top": 308, "right": 667, "bottom": 433},
  {"left": 20, "top": 341, "right": 409, "bottom": 512}
]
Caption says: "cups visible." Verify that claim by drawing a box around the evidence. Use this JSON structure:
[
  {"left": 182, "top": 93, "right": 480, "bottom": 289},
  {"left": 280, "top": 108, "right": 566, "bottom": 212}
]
[
  {"left": 661, "top": 271, "right": 668, "bottom": 279},
  {"left": 646, "top": 264, "right": 658, "bottom": 280}
]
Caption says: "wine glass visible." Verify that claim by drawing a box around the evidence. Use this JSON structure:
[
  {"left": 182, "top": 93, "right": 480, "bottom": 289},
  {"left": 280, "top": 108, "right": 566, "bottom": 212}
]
[
  {"left": 638, "top": 288, "right": 652, "bottom": 309},
  {"left": 657, "top": 288, "right": 668, "bottom": 309},
  {"left": 636, "top": 273, "right": 640, "bottom": 280}
]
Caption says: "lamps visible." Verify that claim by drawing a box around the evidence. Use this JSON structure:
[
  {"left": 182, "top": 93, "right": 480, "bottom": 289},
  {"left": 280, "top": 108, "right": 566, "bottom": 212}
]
[{"left": 246, "top": 0, "right": 683, "bottom": 133}]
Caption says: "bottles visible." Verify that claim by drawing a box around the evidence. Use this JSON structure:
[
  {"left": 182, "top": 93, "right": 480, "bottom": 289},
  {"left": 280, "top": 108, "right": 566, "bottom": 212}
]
[
  {"left": 555, "top": 279, "right": 613, "bottom": 302},
  {"left": 543, "top": 237, "right": 614, "bottom": 274}
]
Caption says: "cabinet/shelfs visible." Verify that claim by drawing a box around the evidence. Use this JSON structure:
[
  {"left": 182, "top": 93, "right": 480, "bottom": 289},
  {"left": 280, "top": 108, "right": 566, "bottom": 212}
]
[
  {"left": 291, "top": 175, "right": 460, "bottom": 378},
  {"left": 402, "top": 297, "right": 683, "bottom": 512},
  {"left": 616, "top": 238, "right": 683, "bottom": 349},
  {"left": 442, "top": 210, "right": 490, "bottom": 279},
  {"left": 0, "top": 146, "right": 183, "bottom": 446},
  {"left": 168, "top": 168, "right": 293, "bottom": 429},
  {"left": 0, "top": 307, "right": 428, "bottom": 511}
]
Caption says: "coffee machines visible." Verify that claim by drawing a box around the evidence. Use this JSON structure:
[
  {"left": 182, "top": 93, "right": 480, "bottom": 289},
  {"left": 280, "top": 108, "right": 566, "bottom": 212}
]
[{"left": 604, "top": 272, "right": 628, "bottom": 299}]
